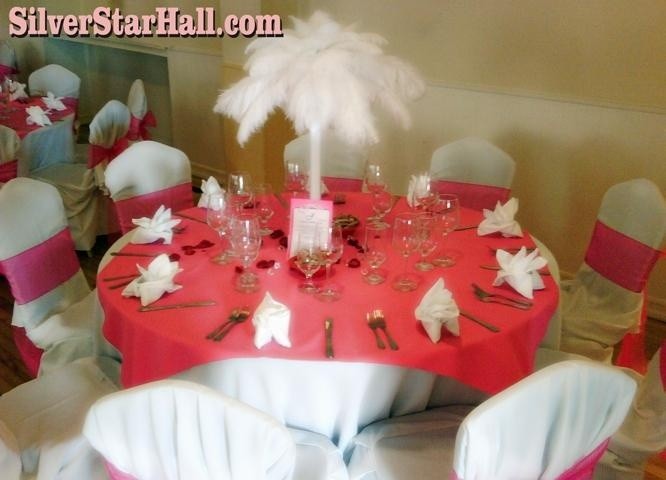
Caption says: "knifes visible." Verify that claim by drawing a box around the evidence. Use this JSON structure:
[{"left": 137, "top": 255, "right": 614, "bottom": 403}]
[
  {"left": 459, "top": 307, "right": 499, "bottom": 333},
  {"left": 324, "top": 314, "right": 334, "bottom": 359},
  {"left": 172, "top": 212, "right": 208, "bottom": 224},
  {"left": 454, "top": 225, "right": 478, "bottom": 231},
  {"left": 110, "top": 251, "right": 158, "bottom": 258},
  {"left": 137, "top": 300, "right": 216, "bottom": 313},
  {"left": 480, "top": 263, "right": 501, "bottom": 270}
]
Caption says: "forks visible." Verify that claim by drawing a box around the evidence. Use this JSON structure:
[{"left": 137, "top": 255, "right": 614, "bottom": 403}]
[
  {"left": 367, "top": 311, "right": 385, "bottom": 351},
  {"left": 472, "top": 283, "right": 534, "bottom": 313},
  {"left": 485, "top": 244, "right": 539, "bottom": 253},
  {"left": 372, "top": 308, "right": 401, "bottom": 350}
]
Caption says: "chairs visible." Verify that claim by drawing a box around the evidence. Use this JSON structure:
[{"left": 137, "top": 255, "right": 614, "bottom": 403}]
[{"left": 0, "top": 41, "right": 666, "bottom": 480}]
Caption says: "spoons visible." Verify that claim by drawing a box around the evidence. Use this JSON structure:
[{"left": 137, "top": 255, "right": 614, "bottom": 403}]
[{"left": 205, "top": 306, "right": 249, "bottom": 341}]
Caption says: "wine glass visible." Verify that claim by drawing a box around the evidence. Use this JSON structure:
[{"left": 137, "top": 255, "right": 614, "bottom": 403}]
[{"left": 204, "top": 156, "right": 463, "bottom": 300}]
